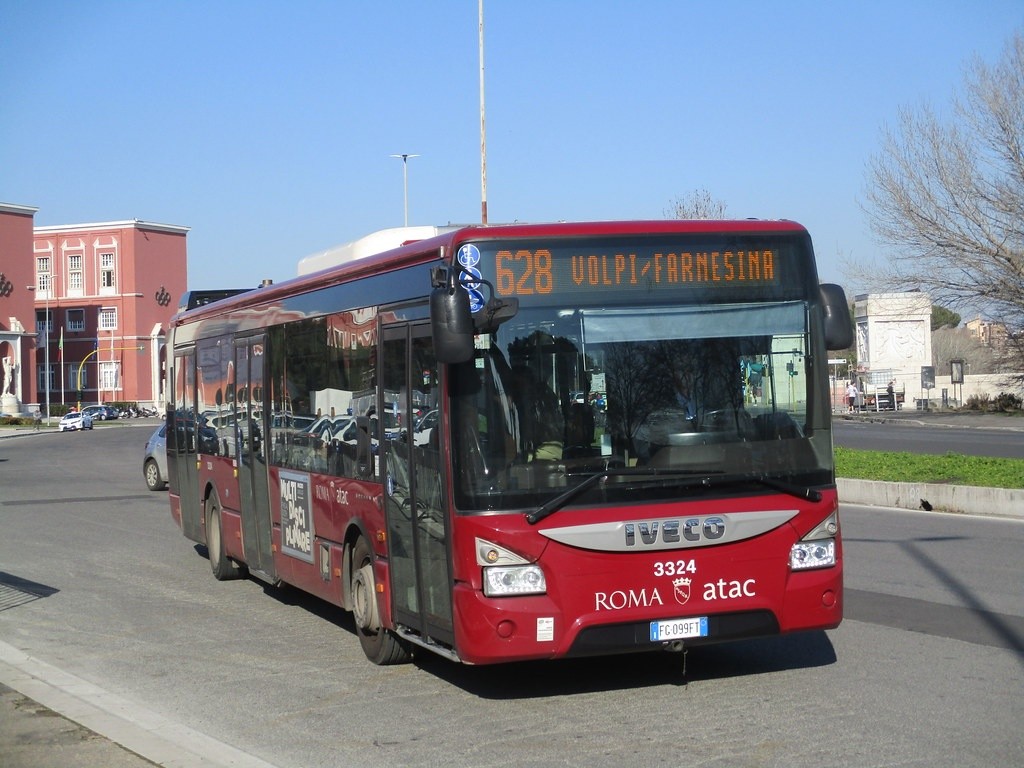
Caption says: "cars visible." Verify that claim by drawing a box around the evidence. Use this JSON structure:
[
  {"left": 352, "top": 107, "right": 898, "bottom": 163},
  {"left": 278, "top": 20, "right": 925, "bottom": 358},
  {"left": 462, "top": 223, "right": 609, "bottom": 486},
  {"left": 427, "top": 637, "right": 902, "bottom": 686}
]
[
  {"left": 143, "top": 421, "right": 170, "bottom": 490},
  {"left": 58, "top": 411, "right": 93, "bottom": 432},
  {"left": 574, "top": 391, "right": 607, "bottom": 413},
  {"left": 413, "top": 408, "right": 438, "bottom": 447}
]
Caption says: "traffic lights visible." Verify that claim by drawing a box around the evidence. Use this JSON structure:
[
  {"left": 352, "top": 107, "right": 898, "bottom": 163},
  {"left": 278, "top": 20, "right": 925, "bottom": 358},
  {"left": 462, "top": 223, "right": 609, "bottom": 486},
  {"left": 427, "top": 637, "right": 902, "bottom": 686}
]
[
  {"left": 77, "top": 390, "right": 82, "bottom": 401},
  {"left": 790, "top": 370, "right": 798, "bottom": 375}
]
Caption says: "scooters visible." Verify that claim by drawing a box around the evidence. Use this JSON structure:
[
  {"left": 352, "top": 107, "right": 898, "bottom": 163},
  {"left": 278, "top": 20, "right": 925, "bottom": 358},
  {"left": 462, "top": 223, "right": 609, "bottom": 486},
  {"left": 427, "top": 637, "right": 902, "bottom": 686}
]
[{"left": 116, "top": 404, "right": 159, "bottom": 419}]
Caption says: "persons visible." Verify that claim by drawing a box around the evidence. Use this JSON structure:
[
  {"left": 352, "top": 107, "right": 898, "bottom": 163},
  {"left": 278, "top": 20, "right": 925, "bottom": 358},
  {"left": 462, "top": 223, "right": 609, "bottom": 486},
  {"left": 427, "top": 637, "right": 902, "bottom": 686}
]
[
  {"left": 648, "top": 350, "right": 741, "bottom": 440},
  {"left": 2, "top": 356, "right": 16, "bottom": 397},
  {"left": 746, "top": 382, "right": 758, "bottom": 406},
  {"left": 33, "top": 408, "right": 42, "bottom": 431},
  {"left": 589, "top": 392, "right": 607, "bottom": 426},
  {"left": 68, "top": 407, "right": 77, "bottom": 413},
  {"left": 884, "top": 381, "right": 896, "bottom": 410},
  {"left": 845, "top": 381, "right": 859, "bottom": 415},
  {"left": 474, "top": 378, "right": 562, "bottom": 465}
]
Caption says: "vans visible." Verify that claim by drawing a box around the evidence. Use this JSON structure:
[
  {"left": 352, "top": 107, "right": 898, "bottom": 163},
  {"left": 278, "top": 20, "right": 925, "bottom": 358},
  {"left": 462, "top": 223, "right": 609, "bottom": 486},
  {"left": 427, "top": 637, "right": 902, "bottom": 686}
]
[
  {"left": 81, "top": 405, "right": 119, "bottom": 420},
  {"left": 202, "top": 400, "right": 274, "bottom": 450}
]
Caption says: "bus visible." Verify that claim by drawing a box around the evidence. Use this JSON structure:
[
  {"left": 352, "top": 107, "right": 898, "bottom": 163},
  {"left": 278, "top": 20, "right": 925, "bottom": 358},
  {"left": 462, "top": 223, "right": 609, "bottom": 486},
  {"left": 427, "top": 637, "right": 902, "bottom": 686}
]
[{"left": 166, "top": 219, "right": 856, "bottom": 668}]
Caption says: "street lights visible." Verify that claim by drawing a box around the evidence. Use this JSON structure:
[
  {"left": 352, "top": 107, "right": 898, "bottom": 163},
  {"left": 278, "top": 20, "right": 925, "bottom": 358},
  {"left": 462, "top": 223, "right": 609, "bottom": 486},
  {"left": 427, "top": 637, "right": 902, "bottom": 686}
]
[
  {"left": 35, "top": 275, "right": 59, "bottom": 426},
  {"left": 389, "top": 153, "right": 419, "bottom": 227}
]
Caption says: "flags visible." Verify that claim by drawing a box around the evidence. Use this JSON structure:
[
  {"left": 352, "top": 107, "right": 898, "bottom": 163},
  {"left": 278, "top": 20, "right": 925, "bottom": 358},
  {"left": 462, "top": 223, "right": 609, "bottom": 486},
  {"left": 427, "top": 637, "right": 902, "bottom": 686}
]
[
  {"left": 91, "top": 338, "right": 98, "bottom": 360},
  {"left": 57, "top": 328, "right": 63, "bottom": 363}
]
